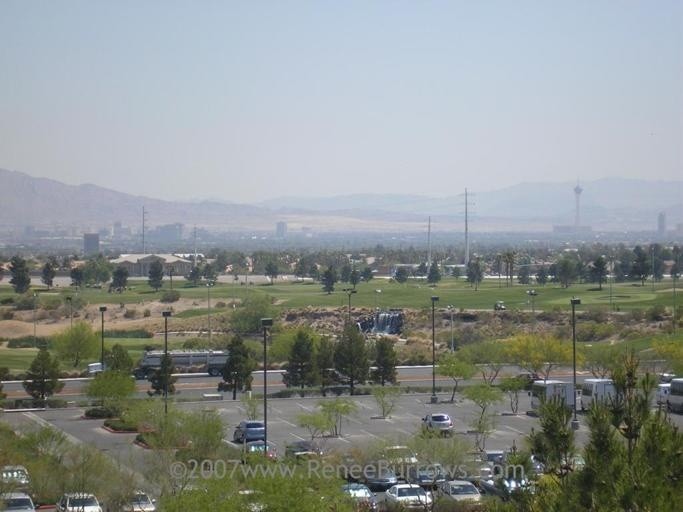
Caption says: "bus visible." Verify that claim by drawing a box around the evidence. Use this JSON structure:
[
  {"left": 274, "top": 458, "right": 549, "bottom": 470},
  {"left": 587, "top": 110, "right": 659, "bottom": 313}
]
[{"left": 668, "top": 378, "right": 682, "bottom": 415}]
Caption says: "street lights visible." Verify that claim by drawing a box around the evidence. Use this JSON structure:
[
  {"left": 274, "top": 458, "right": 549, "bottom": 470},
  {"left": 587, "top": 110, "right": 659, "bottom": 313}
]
[
  {"left": 342, "top": 287, "right": 356, "bottom": 325},
  {"left": 261, "top": 318, "right": 273, "bottom": 458},
  {"left": 430, "top": 296, "right": 440, "bottom": 405},
  {"left": 526, "top": 289, "right": 539, "bottom": 329},
  {"left": 100, "top": 307, "right": 106, "bottom": 374},
  {"left": 569, "top": 295, "right": 582, "bottom": 432},
  {"left": 161, "top": 311, "right": 172, "bottom": 414}
]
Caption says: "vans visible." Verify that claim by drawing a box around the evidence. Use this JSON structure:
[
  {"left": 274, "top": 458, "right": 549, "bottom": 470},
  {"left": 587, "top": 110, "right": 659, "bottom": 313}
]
[{"left": 656, "top": 383, "right": 671, "bottom": 405}]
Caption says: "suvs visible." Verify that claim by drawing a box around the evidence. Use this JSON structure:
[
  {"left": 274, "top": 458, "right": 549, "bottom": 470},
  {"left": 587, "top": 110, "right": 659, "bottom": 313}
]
[{"left": 0, "top": 464, "right": 35, "bottom": 491}]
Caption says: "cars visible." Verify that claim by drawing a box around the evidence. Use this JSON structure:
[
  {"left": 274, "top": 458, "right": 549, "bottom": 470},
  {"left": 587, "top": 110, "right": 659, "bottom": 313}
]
[
  {"left": 107, "top": 486, "right": 156, "bottom": 512},
  {"left": 242, "top": 441, "right": 279, "bottom": 459},
  {"left": 340, "top": 449, "right": 586, "bottom": 512},
  {"left": 54, "top": 491, "right": 103, "bottom": 512},
  {"left": 421, "top": 414, "right": 453, "bottom": 439},
  {"left": 233, "top": 420, "right": 267, "bottom": 443},
  {"left": 284, "top": 441, "right": 324, "bottom": 461},
  {"left": 0, "top": 492, "right": 39, "bottom": 512},
  {"left": 238, "top": 488, "right": 269, "bottom": 512}
]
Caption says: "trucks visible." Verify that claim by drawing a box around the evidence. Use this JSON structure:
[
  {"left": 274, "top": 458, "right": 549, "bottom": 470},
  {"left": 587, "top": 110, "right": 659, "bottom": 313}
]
[
  {"left": 527, "top": 380, "right": 575, "bottom": 410},
  {"left": 581, "top": 380, "right": 624, "bottom": 412},
  {"left": 132, "top": 348, "right": 233, "bottom": 379}
]
